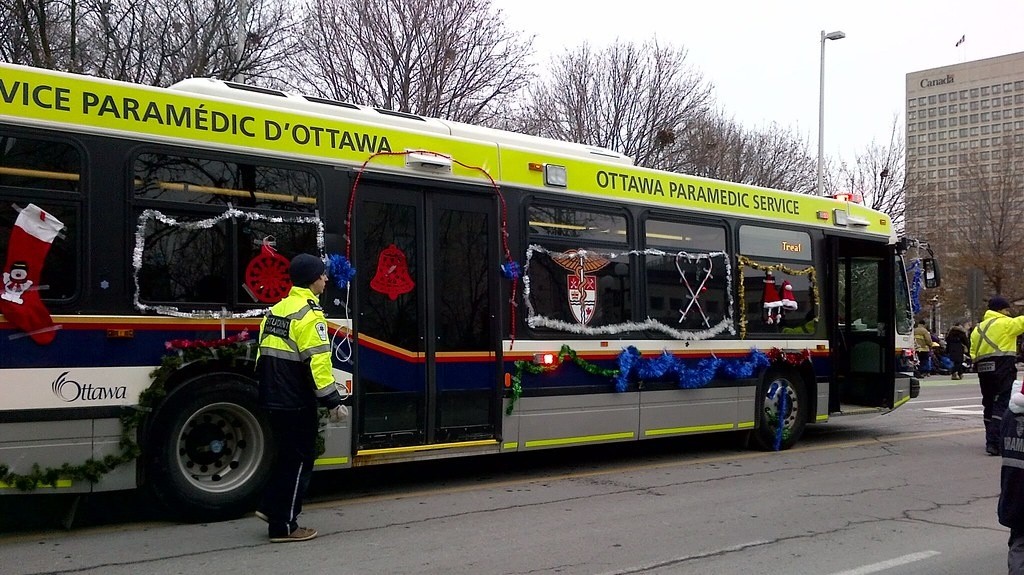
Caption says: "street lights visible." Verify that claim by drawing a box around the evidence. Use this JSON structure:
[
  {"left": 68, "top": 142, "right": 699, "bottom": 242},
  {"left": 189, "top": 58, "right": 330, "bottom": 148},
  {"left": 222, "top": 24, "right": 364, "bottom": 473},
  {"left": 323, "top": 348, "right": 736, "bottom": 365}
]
[{"left": 818, "top": 29, "right": 845, "bottom": 197}]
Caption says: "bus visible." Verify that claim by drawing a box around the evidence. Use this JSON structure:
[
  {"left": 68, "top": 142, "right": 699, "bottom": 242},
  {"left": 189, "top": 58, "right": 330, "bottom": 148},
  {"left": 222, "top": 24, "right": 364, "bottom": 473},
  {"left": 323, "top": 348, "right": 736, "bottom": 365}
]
[{"left": 0, "top": 61, "right": 941, "bottom": 495}]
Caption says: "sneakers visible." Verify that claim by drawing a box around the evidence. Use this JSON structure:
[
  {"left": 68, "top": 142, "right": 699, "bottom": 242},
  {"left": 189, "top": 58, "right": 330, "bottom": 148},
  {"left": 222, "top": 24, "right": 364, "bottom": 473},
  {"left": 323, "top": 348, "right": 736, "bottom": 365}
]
[
  {"left": 268, "top": 521, "right": 317, "bottom": 543},
  {"left": 255, "top": 506, "right": 268, "bottom": 522}
]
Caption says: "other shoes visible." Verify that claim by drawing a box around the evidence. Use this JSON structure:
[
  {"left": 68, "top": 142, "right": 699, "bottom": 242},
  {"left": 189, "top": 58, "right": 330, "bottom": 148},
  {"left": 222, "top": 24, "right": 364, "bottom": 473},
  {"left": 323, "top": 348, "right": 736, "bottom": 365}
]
[
  {"left": 986, "top": 442, "right": 1001, "bottom": 455},
  {"left": 951, "top": 373, "right": 962, "bottom": 380},
  {"left": 917, "top": 371, "right": 924, "bottom": 378}
]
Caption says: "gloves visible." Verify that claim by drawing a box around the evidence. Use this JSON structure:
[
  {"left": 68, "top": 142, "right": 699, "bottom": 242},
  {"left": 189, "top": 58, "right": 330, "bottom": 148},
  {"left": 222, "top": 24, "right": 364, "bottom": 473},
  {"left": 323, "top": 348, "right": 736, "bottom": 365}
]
[{"left": 328, "top": 406, "right": 349, "bottom": 423}]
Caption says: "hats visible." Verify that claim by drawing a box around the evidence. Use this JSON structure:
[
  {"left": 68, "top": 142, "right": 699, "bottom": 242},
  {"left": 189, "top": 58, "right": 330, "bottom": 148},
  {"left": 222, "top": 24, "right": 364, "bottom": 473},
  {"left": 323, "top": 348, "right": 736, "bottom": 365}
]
[
  {"left": 780, "top": 281, "right": 798, "bottom": 310},
  {"left": 763, "top": 269, "right": 783, "bottom": 324},
  {"left": 988, "top": 298, "right": 1010, "bottom": 311},
  {"left": 289, "top": 253, "right": 325, "bottom": 287}
]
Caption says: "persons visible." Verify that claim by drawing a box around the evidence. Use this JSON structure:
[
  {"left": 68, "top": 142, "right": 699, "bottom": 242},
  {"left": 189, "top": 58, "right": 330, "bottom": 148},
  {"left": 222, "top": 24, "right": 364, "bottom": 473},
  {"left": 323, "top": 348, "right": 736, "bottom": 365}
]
[
  {"left": 998, "top": 371, "right": 1024, "bottom": 575},
  {"left": 942, "top": 320, "right": 970, "bottom": 380},
  {"left": 913, "top": 320, "right": 936, "bottom": 378},
  {"left": 246, "top": 254, "right": 350, "bottom": 541},
  {"left": 969, "top": 296, "right": 1024, "bottom": 457}
]
